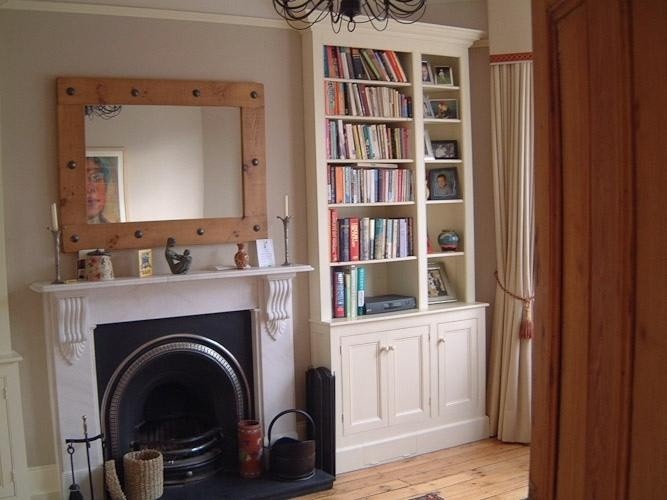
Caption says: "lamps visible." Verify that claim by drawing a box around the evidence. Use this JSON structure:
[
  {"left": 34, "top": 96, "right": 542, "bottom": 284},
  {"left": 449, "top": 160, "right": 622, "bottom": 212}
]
[{"left": 268, "top": 1, "right": 430, "bottom": 34}]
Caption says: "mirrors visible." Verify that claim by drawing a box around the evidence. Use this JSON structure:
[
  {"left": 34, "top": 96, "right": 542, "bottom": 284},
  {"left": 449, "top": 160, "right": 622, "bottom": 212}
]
[{"left": 54, "top": 75, "right": 268, "bottom": 256}]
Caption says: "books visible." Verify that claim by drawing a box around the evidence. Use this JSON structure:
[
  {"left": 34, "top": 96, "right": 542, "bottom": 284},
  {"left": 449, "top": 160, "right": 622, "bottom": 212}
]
[{"left": 322, "top": 45, "right": 415, "bottom": 318}]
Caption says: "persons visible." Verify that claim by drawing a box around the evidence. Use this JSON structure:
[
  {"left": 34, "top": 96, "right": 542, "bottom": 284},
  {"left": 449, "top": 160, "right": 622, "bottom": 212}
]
[
  {"left": 435, "top": 144, "right": 448, "bottom": 158},
  {"left": 84, "top": 154, "right": 110, "bottom": 226},
  {"left": 422, "top": 62, "right": 430, "bottom": 81},
  {"left": 428, "top": 271, "right": 441, "bottom": 296},
  {"left": 439, "top": 68, "right": 445, "bottom": 79},
  {"left": 435, "top": 175, "right": 452, "bottom": 195}
]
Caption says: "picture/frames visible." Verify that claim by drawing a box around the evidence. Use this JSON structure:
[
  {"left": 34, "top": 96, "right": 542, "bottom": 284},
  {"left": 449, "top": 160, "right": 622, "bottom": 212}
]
[
  {"left": 85, "top": 144, "right": 129, "bottom": 225},
  {"left": 137, "top": 248, "right": 152, "bottom": 278},
  {"left": 422, "top": 60, "right": 459, "bottom": 160},
  {"left": 425, "top": 261, "right": 458, "bottom": 305},
  {"left": 427, "top": 167, "right": 460, "bottom": 202}
]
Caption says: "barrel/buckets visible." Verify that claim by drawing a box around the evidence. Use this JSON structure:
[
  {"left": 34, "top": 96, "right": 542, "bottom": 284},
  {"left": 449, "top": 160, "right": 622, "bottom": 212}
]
[{"left": 267, "top": 410, "right": 317, "bottom": 482}]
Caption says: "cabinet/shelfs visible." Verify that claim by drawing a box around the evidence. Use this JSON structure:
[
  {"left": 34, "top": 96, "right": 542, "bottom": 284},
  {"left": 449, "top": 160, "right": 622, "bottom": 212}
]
[
  {"left": 417, "top": 53, "right": 475, "bottom": 306},
  {"left": 304, "top": 40, "right": 424, "bottom": 323},
  {"left": 308, "top": 315, "right": 434, "bottom": 475},
  {"left": 432, "top": 304, "right": 491, "bottom": 451}
]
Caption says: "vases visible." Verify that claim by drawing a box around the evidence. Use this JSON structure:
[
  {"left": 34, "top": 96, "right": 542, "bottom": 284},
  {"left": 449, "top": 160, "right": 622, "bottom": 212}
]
[
  {"left": 235, "top": 419, "right": 263, "bottom": 479},
  {"left": 234, "top": 242, "right": 249, "bottom": 270},
  {"left": 437, "top": 229, "right": 461, "bottom": 251}
]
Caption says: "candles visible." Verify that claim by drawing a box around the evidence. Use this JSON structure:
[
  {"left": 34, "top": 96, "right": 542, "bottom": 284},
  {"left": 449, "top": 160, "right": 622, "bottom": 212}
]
[
  {"left": 49, "top": 200, "right": 60, "bottom": 232},
  {"left": 283, "top": 194, "right": 290, "bottom": 218}
]
